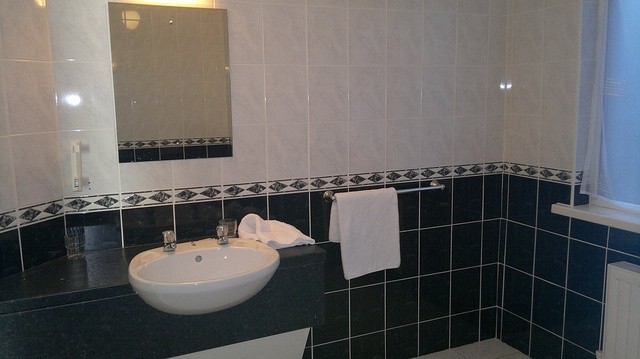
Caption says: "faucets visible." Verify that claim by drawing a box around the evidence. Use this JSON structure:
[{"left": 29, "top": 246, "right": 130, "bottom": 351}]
[
  {"left": 216, "top": 225, "right": 229, "bottom": 245},
  {"left": 162, "top": 230, "right": 177, "bottom": 253}
]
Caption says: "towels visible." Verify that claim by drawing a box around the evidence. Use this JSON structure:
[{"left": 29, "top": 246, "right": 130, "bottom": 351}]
[
  {"left": 238, "top": 213, "right": 316, "bottom": 252},
  {"left": 328, "top": 186, "right": 403, "bottom": 281}
]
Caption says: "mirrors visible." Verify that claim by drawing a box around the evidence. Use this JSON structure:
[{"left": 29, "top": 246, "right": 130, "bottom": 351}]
[{"left": 107, "top": 0, "right": 233, "bottom": 164}]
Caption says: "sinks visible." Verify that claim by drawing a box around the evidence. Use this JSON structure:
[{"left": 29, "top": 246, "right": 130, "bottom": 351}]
[{"left": 127, "top": 237, "right": 281, "bottom": 317}]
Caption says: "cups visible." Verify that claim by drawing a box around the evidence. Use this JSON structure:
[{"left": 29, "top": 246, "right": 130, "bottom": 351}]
[{"left": 219, "top": 218, "right": 237, "bottom": 238}]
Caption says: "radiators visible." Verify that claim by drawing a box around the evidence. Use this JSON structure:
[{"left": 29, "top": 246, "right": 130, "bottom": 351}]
[{"left": 595, "top": 259, "right": 640, "bottom": 359}]
